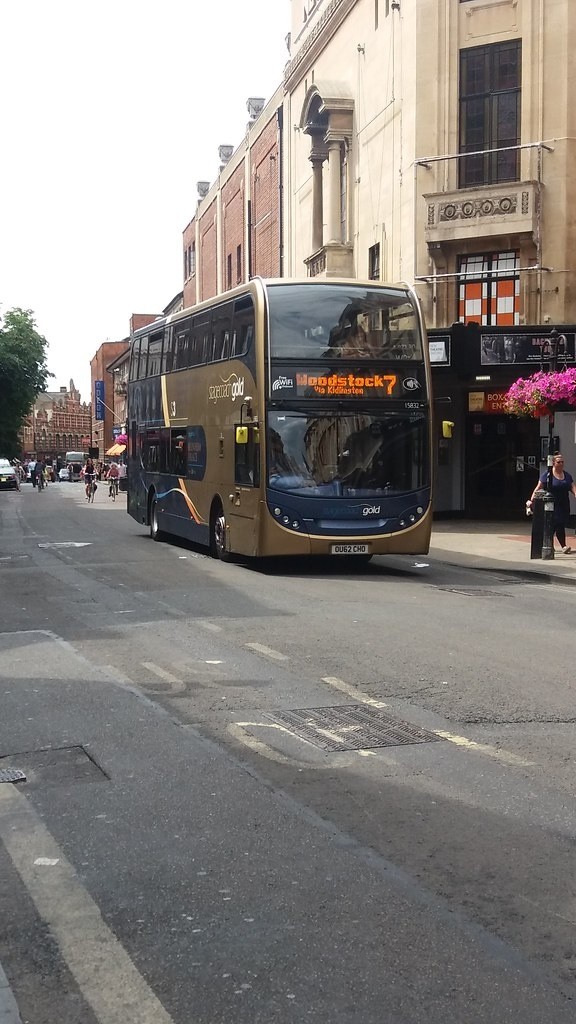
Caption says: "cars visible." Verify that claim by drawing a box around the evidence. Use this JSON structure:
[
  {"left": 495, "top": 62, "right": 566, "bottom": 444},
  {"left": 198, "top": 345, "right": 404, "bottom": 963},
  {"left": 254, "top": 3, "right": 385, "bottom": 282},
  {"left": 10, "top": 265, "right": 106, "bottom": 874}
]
[
  {"left": 57, "top": 468, "right": 70, "bottom": 482},
  {"left": 0, "top": 456, "right": 56, "bottom": 491}
]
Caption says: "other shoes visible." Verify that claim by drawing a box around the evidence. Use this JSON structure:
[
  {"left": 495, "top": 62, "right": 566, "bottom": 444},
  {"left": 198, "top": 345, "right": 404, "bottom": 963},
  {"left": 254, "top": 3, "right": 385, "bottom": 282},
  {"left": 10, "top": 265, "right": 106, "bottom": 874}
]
[{"left": 562, "top": 545, "right": 571, "bottom": 553}]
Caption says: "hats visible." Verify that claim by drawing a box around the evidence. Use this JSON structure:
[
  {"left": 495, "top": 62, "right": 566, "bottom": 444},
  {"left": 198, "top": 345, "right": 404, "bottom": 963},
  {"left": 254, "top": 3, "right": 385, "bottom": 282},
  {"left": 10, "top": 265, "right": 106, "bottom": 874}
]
[
  {"left": 111, "top": 463, "right": 117, "bottom": 468},
  {"left": 119, "top": 459, "right": 123, "bottom": 462}
]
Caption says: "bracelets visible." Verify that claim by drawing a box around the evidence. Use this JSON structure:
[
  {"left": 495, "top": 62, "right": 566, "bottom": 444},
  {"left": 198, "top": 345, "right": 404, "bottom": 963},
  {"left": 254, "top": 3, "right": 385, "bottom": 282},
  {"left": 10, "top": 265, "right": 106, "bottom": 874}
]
[{"left": 531, "top": 500, "right": 533, "bottom": 502}]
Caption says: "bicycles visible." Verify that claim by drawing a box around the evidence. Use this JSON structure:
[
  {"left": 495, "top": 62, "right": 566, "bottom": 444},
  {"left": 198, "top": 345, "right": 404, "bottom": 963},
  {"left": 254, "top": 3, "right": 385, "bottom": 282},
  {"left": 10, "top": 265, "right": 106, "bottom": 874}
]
[
  {"left": 84, "top": 473, "right": 96, "bottom": 503},
  {"left": 107, "top": 476, "right": 119, "bottom": 502}
]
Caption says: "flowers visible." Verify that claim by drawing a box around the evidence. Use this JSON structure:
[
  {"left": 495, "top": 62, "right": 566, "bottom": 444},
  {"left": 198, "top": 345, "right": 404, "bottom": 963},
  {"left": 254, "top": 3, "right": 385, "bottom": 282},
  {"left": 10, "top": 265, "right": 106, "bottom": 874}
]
[{"left": 503, "top": 368, "right": 576, "bottom": 418}]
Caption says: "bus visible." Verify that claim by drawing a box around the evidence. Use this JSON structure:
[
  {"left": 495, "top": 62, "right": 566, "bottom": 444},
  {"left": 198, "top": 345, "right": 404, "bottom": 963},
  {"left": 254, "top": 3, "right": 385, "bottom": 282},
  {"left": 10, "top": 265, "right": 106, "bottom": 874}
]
[
  {"left": 65, "top": 451, "right": 89, "bottom": 482},
  {"left": 121, "top": 274, "right": 456, "bottom": 563}
]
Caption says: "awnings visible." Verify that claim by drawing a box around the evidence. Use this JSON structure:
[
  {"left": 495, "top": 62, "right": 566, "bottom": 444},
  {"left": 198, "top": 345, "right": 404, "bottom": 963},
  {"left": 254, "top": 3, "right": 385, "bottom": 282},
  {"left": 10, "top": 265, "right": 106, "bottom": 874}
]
[{"left": 106, "top": 442, "right": 127, "bottom": 456}]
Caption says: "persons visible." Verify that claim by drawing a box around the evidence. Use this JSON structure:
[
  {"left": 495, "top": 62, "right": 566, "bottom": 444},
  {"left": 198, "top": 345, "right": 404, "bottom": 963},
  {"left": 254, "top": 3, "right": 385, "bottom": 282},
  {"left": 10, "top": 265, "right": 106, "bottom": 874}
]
[
  {"left": 11, "top": 456, "right": 126, "bottom": 500},
  {"left": 330, "top": 328, "right": 377, "bottom": 360},
  {"left": 530, "top": 450, "right": 576, "bottom": 554},
  {"left": 267, "top": 441, "right": 316, "bottom": 489}
]
